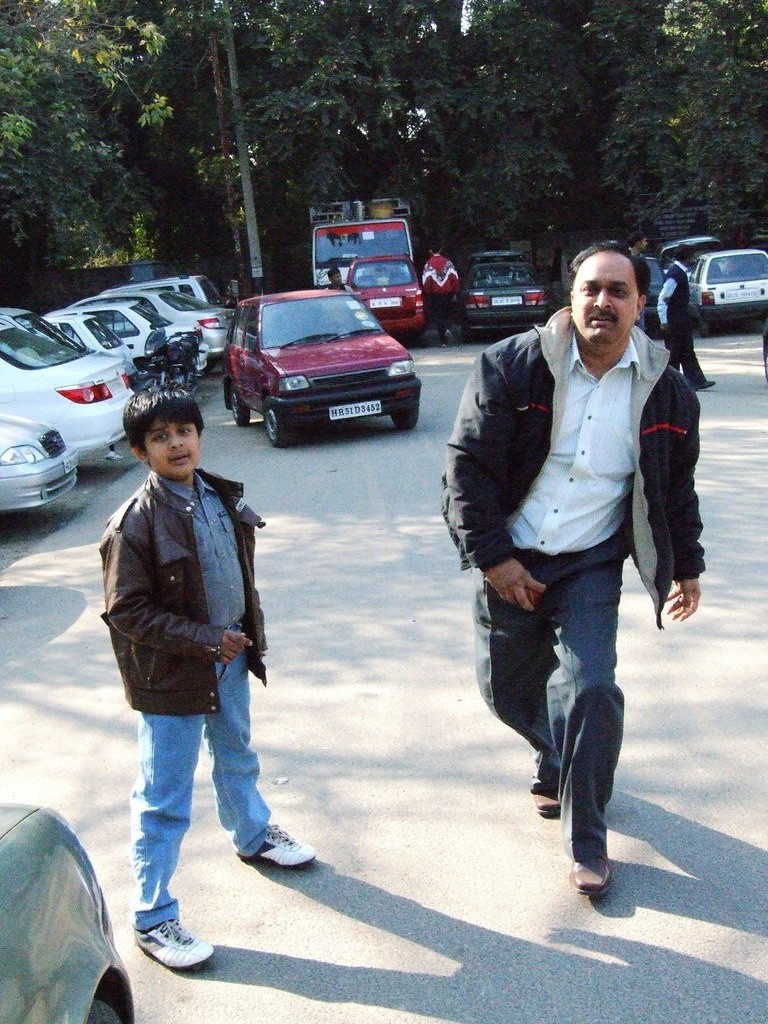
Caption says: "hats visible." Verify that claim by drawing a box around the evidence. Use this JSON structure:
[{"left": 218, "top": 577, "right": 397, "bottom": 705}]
[{"left": 674, "top": 243, "right": 700, "bottom": 260}]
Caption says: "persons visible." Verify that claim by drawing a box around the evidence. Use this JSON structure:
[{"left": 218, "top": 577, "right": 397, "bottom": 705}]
[
  {"left": 324, "top": 268, "right": 353, "bottom": 293},
  {"left": 627, "top": 229, "right": 717, "bottom": 392},
  {"left": 421, "top": 243, "right": 461, "bottom": 349},
  {"left": 442, "top": 242, "right": 709, "bottom": 898},
  {"left": 94, "top": 384, "right": 319, "bottom": 975}
]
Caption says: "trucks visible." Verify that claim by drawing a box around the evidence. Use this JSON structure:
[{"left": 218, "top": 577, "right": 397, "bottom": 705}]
[{"left": 308, "top": 195, "right": 414, "bottom": 287}]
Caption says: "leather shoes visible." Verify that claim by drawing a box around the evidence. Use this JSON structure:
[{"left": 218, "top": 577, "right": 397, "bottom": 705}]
[
  {"left": 569, "top": 843, "right": 612, "bottom": 894},
  {"left": 534, "top": 789, "right": 561, "bottom": 816}
]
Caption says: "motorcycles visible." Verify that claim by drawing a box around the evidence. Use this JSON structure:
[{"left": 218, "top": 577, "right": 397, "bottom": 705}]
[{"left": 144, "top": 328, "right": 202, "bottom": 402}]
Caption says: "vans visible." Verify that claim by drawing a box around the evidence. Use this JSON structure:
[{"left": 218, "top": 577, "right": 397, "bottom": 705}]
[{"left": 346, "top": 254, "right": 425, "bottom": 342}]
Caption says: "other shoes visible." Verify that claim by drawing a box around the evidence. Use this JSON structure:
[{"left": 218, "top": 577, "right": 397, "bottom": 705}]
[
  {"left": 444, "top": 330, "right": 454, "bottom": 344},
  {"left": 693, "top": 380, "right": 716, "bottom": 391}
]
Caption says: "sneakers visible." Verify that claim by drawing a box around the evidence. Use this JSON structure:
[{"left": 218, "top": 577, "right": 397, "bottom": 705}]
[
  {"left": 256, "top": 824, "right": 316, "bottom": 866},
  {"left": 135, "top": 919, "right": 214, "bottom": 967}
]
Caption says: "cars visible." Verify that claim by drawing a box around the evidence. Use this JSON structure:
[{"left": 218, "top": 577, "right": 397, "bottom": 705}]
[
  {"left": 0, "top": 273, "right": 233, "bottom": 512},
  {"left": 222, "top": 291, "right": 420, "bottom": 448},
  {"left": 462, "top": 261, "right": 547, "bottom": 334},
  {"left": 641, "top": 234, "right": 768, "bottom": 338}
]
